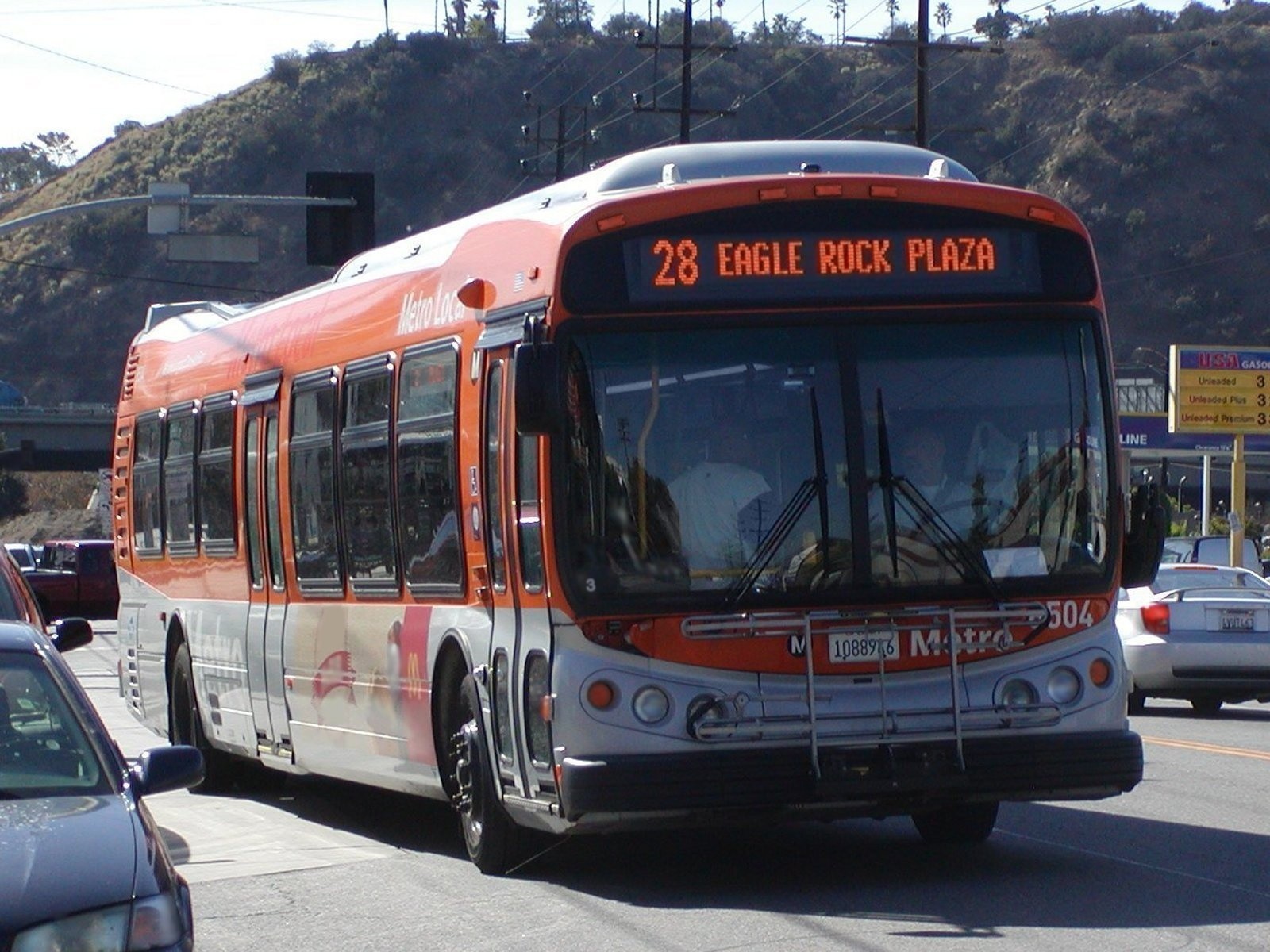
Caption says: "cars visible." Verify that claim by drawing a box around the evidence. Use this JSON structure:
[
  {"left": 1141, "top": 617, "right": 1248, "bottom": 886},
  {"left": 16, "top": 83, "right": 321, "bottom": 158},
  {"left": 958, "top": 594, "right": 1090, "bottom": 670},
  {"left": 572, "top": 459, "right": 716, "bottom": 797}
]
[
  {"left": 0, "top": 539, "right": 207, "bottom": 952},
  {"left": 1117, "top": 534, "right": 1270, "bottom": 717}
]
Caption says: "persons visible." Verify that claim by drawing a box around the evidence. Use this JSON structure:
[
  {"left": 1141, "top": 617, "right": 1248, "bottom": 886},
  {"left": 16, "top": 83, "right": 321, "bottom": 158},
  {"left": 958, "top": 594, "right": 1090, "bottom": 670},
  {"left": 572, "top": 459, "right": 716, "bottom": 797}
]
[
  {"left": 658, "top": 417, "right": 790, "bottom": 612},
  {"left": 866, "top": 420, "right": 994, "bottom": 539}
]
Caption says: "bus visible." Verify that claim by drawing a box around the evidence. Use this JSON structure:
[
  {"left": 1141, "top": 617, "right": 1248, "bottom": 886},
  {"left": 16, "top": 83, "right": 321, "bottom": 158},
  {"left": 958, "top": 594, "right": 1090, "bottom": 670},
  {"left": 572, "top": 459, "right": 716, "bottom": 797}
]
[
  {"left": 109, "top": 140, "right": 1168, "bottom": 876},
  {"left": 131, "top": 411, "right": 545, "bottom": 583}
]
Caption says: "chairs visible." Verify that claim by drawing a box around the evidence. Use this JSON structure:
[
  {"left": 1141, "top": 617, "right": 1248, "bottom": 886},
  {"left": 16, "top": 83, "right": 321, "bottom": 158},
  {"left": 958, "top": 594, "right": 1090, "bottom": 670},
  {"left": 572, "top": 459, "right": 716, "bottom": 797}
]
[{"left": 0, "top": 685, "right": 22, "bottom": 739}]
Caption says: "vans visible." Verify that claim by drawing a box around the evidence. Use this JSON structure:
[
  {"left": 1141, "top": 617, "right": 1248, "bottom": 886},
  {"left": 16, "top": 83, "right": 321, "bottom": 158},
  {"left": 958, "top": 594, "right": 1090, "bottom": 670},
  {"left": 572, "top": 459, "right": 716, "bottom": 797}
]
[{"left": 0, "top": 380, "right": 30, "bottom": 416}]
